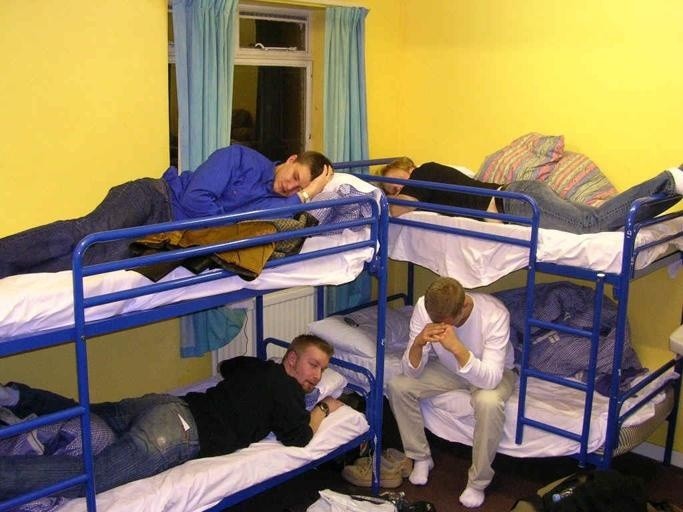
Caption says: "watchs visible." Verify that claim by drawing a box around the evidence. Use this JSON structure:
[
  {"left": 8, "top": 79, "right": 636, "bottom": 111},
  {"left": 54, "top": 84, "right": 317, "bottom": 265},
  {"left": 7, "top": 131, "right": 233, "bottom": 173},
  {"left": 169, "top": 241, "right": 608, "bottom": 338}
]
[{"left": 313, "top": 402, "right": 329, "bottom": 417}]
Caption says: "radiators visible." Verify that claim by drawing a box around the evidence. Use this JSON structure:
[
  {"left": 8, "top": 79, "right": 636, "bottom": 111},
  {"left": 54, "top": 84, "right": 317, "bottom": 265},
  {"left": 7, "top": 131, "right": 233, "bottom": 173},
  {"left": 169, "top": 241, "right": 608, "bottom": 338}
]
[{"left": 213, "top": 283, "right": 329, "bottom": 382}]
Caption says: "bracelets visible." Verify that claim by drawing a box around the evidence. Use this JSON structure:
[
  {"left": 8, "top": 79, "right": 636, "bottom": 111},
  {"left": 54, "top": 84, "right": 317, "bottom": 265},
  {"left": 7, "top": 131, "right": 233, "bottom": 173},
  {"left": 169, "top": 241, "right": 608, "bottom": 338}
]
[{"left": 299, "top": 189, "right": 310, "bottom": 204}]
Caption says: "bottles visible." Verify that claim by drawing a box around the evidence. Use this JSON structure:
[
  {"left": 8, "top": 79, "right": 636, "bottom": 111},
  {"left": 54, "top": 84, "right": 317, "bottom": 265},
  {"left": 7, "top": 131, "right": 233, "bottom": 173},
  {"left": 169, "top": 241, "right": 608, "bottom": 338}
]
[{"left": 553, "top": 486, "right": 577, "bottom": 502}]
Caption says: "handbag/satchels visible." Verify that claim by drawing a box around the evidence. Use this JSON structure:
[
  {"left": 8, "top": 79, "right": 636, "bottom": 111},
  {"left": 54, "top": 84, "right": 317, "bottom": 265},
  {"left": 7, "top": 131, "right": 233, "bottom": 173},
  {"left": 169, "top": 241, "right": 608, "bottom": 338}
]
[{"left": 540, "top": 468, "right": 646, "bottom": 511}]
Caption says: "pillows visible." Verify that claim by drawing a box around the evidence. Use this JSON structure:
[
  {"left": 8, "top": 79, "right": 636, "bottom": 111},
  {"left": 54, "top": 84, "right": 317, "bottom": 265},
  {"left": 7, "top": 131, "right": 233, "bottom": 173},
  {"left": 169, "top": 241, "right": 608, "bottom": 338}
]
[
  {"left": 321, "top": 172, "right": 381, "bottom": 216},
  {"left": 270, "top": 357, "right": 347, "bottom": 413},
  {"left": 307, "top": 306, "right": 410, "bottom": 359}
]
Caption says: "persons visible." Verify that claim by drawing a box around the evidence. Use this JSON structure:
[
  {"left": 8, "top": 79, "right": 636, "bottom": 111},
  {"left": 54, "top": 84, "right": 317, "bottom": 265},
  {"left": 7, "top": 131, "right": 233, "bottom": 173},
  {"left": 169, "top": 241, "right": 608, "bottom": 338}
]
[
  {"left": 0, "top": 143, "right": 335, "bottom": 279},
  {"left": 386, "top": 276, "right": 517, "bottom": 509},
  {"left": 0, "top": 334, "right": 344, "bottom": 503},
  {"left": 376, "top": 155, "right": 683, "bottom": 234}
]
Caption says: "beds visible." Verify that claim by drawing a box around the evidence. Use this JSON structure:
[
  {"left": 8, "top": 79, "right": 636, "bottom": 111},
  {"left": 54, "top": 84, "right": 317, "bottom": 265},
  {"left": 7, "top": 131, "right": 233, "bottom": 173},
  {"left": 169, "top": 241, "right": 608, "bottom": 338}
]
[
  {"left": 0, "top": 194, "right": 387, "bottom": 512},
  {"left": 308, "top": 156, "right": 683, "bottom": 509}
]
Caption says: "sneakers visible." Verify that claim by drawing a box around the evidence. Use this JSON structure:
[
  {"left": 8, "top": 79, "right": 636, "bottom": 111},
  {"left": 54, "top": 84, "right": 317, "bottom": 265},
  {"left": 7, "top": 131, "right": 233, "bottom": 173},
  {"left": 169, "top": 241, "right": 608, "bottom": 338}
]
[
  {"left": 354, "top": 447, "right": 414, "bottom": 478},
  {"left": 340, "top": 456, "right": 405, "bottom": 489}
]
